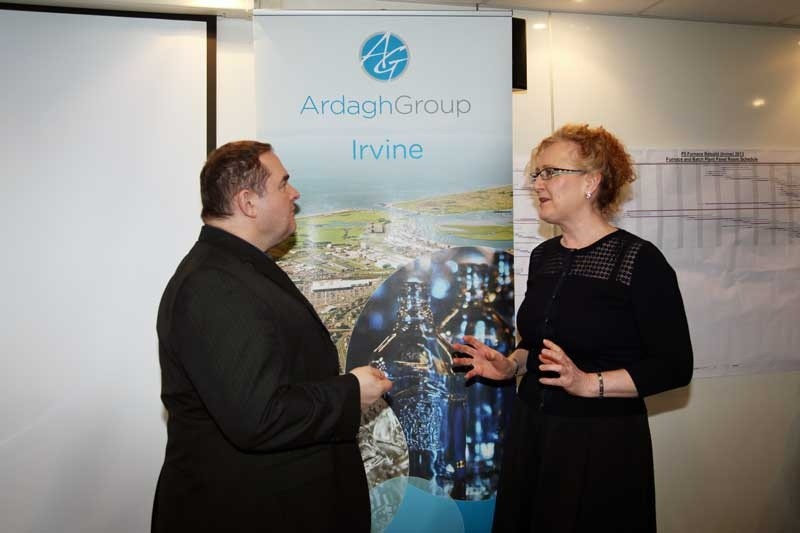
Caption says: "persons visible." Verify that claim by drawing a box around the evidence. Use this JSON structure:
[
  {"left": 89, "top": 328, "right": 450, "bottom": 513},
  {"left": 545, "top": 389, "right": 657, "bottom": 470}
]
[
  {"left": 150, "top": 141, "right": 393, "bottom": 533},
  {"left": 451, "top": 125, "right": 694, "bottom": 533}
]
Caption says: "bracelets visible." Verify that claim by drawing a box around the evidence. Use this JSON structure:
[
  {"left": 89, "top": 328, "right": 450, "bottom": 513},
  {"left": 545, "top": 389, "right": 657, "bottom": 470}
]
[
  {"left": 597, "top": 372, "right": 604, "bottom": 397},
  {"left": 510, "top": 354, "right": 519, "bottom": 378}
]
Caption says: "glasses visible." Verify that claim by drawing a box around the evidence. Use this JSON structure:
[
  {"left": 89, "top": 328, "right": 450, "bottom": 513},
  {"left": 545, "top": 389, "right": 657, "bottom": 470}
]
[{"left": 529, "top": 167, "right": 589, "bottom": 183}]
